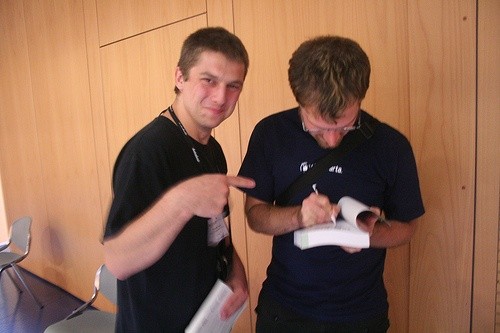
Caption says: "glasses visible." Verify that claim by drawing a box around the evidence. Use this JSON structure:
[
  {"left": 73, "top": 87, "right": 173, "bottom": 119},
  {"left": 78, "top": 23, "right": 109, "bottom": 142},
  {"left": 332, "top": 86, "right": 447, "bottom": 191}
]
[{"left": 301, "top": 107, "right": 362, "bottom": 134}]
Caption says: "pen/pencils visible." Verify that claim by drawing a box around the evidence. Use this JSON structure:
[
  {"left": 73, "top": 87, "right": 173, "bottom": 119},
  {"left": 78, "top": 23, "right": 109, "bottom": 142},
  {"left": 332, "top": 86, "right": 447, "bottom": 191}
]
[{"left": 312, "top": 183, "right": 337, "bottom": 227}]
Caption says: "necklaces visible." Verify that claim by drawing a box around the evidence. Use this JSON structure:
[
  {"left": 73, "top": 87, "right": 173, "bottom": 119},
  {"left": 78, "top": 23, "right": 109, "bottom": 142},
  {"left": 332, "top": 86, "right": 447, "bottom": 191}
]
[{"left": 158, "top": 105, "right": 222, "bottom": 174}]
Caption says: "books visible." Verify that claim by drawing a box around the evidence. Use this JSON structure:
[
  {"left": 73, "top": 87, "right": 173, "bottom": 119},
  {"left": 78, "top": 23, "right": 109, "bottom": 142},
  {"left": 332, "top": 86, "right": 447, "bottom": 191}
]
[
  {"left": 293, "top": 195, "right": 391, "bottom": 250},
  {"left": 184, "top": 278, "right": 248, "bottom": 333}
]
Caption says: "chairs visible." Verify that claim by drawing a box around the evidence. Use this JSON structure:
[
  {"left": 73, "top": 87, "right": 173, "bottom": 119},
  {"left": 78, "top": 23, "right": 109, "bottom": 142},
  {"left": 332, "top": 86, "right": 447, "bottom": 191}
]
[
  {"left": 43, "top": 264, "right": 127, "bottom": 333},
  {"left": 0, "top": 215, "right": 48, "bottom": 310}
]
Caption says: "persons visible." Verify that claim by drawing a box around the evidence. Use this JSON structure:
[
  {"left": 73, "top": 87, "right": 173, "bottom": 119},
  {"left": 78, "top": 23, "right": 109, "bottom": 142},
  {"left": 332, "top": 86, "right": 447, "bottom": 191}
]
[
  {"left": 233, "top": 34, "right": 426, "bottom": 333},
  {"left": 99, "top": 26, "right": 258, "bottom": 333}
]
